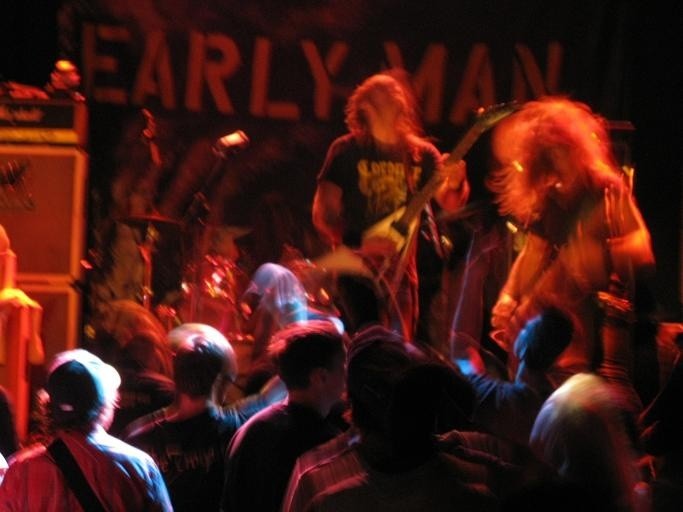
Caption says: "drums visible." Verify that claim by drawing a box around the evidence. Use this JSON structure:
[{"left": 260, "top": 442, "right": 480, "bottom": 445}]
[{"left": 175, "top": 256, "right": 268, "bottom": 377}]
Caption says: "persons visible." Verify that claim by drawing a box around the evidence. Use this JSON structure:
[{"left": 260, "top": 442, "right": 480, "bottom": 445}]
[
  {"left": 312, "top": 72, "right": 470, "bottom": 325},
  {"left": 489, "top": 95, "right": 654, "bottom": 387},
  {"left": 0, "top": 261, "right": 683, "bottom": 512},
  {"left": 113, "top": 107, "right": 162, "bottom": 192}
]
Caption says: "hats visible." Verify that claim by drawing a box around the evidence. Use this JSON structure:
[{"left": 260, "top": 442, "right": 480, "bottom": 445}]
[
  {"left": 46, "top": 348, "right": 122, "bottom": 415},
  {"left": 348, "top": 336, "right": 411, "bottom": 385},
  {"left": 176, "top": 334, "right": 236, "bottom": 382}
]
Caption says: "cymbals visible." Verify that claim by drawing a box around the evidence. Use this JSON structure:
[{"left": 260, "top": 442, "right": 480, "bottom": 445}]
[{"left": 117, "top": 216, "right": 184, "bottom": 227}]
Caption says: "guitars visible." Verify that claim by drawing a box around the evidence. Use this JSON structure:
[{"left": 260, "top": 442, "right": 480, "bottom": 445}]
[{"left": 364, "top": 101, "right": 514, "bottom": 263}]
